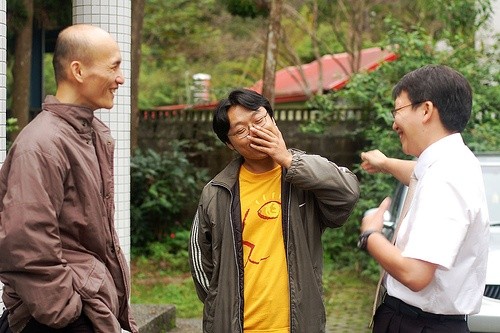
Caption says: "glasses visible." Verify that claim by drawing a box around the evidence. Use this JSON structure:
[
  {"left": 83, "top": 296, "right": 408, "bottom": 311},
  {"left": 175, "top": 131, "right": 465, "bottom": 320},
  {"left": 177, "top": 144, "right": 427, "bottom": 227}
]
[
  {"left": 230, "top": 112, "right": 268, "bottom": 139},
  {"left": 390, "top": 101, "right": 413, "bottom": 119}
]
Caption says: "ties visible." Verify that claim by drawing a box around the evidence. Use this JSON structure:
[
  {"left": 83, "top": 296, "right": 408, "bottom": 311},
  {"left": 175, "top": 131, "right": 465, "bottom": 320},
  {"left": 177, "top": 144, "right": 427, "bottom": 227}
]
[{"left": 368, "top": 171, "right": 418, "bottom": 328}]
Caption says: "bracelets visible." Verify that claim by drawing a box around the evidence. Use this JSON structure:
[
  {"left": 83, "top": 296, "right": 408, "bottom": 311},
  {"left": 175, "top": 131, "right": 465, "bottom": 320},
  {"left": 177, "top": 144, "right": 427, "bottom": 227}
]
[{"left": 358, "top": 229, "right": 385, "bottom": 256}]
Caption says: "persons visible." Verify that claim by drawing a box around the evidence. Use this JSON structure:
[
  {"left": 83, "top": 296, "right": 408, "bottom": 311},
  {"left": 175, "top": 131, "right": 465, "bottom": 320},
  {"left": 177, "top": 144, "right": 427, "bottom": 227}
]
[
  {"left": 0, "top": 25, "right": 139, "bottom": 333},
  {"left": 359, "top": 65, "right": 489, "bottom": 333},
  {"left": 188, "top": 90, "right": 361, "bottom": 333}
]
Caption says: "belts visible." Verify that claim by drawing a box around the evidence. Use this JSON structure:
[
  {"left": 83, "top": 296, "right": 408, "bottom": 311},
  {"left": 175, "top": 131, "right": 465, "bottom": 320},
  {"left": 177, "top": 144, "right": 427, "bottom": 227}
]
[{"left": 382, "top": 292, "right": 466, "bottom": 321}]
